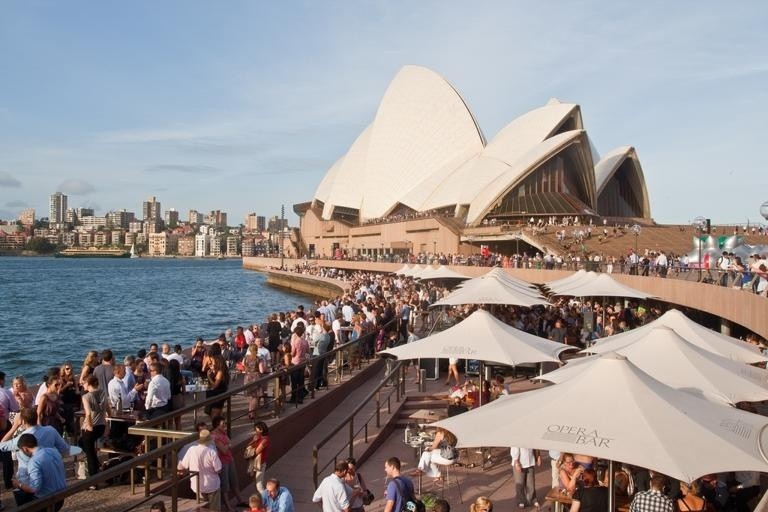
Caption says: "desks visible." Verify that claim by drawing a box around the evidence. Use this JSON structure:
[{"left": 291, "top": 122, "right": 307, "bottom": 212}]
[
  {"left": 546, "top": 485, "right": 632, "bottom": 511},
  {"left": 401, "top": 438, "right": 434, "bottom": 495},
  {"left": 408, "top": 409, "right": 442, "bottom": 423},
  {"left": 74, "top": 410, "right": 144, "bottom": 462},
  {"left": 180, "top": 385, "right": 214, "bottom": 427}
]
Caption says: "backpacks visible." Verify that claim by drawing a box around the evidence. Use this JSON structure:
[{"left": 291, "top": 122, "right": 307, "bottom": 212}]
[{"left": 401, "top": 491, "right": 426, "bottom": 512}]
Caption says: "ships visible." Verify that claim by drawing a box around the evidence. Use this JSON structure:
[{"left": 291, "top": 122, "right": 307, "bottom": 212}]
[{"left": 54, "top": 244, "right": 131, "bottom": 259}]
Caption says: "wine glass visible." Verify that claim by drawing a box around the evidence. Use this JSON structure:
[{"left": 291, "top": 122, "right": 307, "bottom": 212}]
[{"left": 128, "top": 402, "right": 135, "bottom": 417}]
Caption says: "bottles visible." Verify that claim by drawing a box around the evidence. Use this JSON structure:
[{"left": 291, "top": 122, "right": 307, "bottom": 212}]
[
  {"left": 115, "top": 392, "right": 123, "bottom": 418},
  {"left": 404, "top": 421, "right": 412, "bottom": 443},
  {"left": 62, "top": 425, "right": 68, "bottom": 442}
]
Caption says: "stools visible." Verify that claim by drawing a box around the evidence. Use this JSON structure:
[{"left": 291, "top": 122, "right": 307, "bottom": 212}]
[{"left": 432, "top": 464, "right": 463, "bottom": 504}]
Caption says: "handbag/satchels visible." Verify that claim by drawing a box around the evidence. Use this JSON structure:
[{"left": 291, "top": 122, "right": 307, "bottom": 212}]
[
  {"left": 74, "top": 457, "right": 89, "bottom": 480},
  {"left": 357, "top": 472, "right": 375, "bottom": 505}
]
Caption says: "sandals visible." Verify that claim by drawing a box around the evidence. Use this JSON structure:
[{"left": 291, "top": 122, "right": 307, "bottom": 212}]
[
  {"left": 432, "top": 476, "right": 444, "bottom": 482},
  {"left": 409, "top": 470, "right": 422, "bottom": 476}
]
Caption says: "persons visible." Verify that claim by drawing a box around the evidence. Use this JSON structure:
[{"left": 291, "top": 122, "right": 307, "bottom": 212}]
[{"left": 1, "top": 210, "right": 767, "bottom": 511}]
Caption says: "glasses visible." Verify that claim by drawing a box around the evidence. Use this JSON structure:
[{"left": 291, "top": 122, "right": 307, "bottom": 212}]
[
  {"left": 49, "top": 384, "right": 60, "bottom": 387},
  {"left": 65, "top": 368, "right": 70, "bottom": 370}
]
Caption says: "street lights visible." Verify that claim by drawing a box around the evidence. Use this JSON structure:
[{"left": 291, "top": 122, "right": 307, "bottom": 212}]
[
  {"left": 759, "top": 200, "right": 767, "bottom": 221},
  {"left": 521, "top": 211, "right": 525, "bottom": 224},
  {"left": 630, "top": 224, "right": 640, "bottom": 275},
  {"left": 513, "top": 229, "right": 522, "bottom": 267},
  {"left": 691, "top": 216, "right": 706, "bottom": 282}
]
